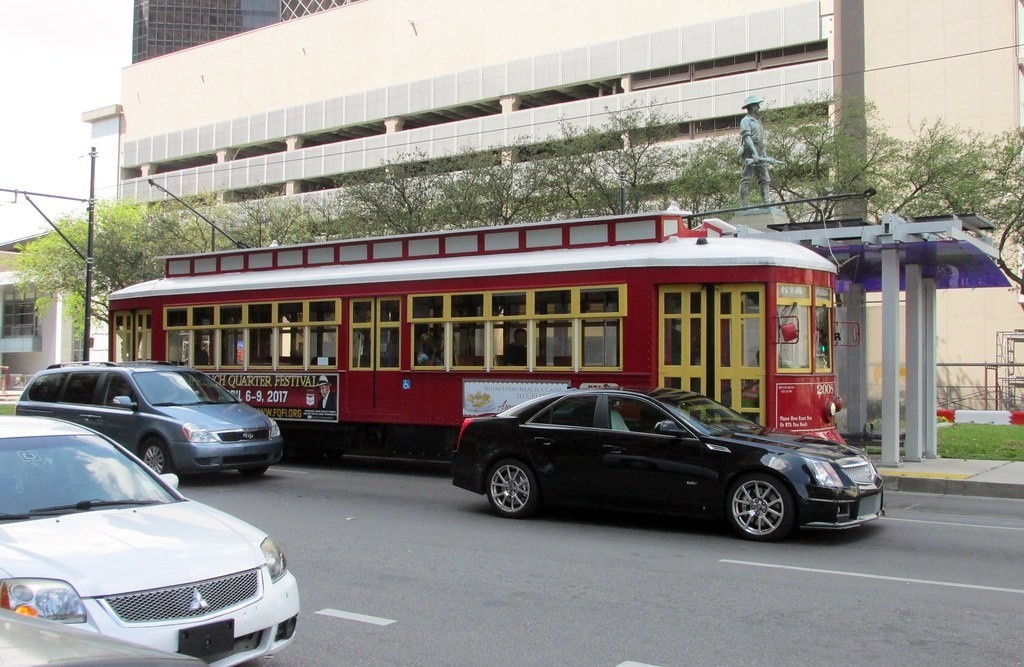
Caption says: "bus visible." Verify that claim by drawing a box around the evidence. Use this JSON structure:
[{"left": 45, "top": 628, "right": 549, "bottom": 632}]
[{"left": 104, "top": 178, "right": 848, "bottom": 465}]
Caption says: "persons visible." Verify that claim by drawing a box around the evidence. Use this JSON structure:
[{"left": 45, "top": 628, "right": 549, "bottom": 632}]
[
  {"left": 737, "top": 95, "right": 775, "bottom": 208},
  {"left": 194, "top": 334, "right": 209, "bottom": 364},
  {"left": 417, "top": 332, "right": 440, "bottom": 366},
  {"left": 316, "top": 375, "right": 337, "bottom": 410},
  {"left": 504, "top": 328, "right": 527, "bottom": 366}
]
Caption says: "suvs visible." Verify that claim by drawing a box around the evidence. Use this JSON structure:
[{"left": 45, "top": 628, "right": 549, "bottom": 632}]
[{"left": 16, "top": 360, "right": 284, "bottom": 481}]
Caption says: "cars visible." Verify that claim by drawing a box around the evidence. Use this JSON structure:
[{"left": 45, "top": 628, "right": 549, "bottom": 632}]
[
  {"left": 0, "top": 413, "right": 301, "bottom": 667},
  {"left": 452, "top": 385, "right": 886, "bottom": 544}
]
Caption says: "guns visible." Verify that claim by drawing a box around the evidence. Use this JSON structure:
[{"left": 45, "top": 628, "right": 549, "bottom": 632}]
[{"left": 744, "top": 156, "right": 786, "bottom": 166}]
